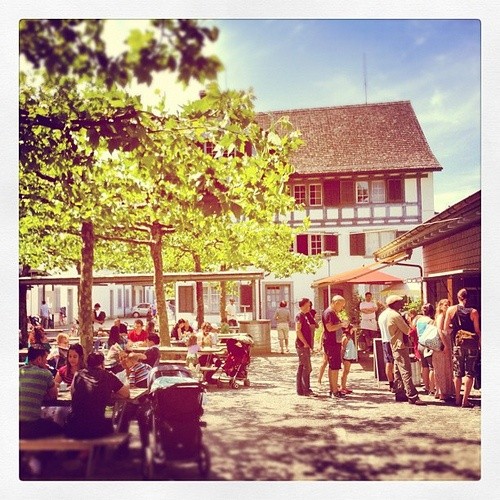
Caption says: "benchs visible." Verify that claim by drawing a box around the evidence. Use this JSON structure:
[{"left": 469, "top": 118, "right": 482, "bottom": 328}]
[
  {"left": 19, "top": 436, "right": 129, "bottom": 481},
  {"left": 159, "top": 359, "right": 218, "bottom": 377}
]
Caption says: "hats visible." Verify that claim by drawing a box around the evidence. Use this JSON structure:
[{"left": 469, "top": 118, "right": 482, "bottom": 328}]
[
  {"left": 56, "top": 334, "right": 70, "bottom": 349},
  {"left": 386, "top": 295, "right": 403, "bottom": 305}
]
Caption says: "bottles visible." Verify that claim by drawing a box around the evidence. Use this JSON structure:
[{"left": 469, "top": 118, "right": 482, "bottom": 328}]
[
  {"left": 202, "top": 337, "right": 204, "bottom": 348},
  {"left": 129, "top": 368, "right": 135, "bottom": 389}
]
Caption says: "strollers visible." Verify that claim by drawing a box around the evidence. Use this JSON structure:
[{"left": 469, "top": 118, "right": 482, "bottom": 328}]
[
  {"left": 141, "top": 365, "right": 211, "bottom": 478},
  {"left": 215, "top": 333, "right": 254, "bottom": 388}
]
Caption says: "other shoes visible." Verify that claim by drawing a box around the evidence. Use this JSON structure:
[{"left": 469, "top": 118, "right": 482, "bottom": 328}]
[
  {"left": 340, "top": 388, "right": 352, "bottom": 394},
  {"left": 334, "top": 392, "right": 347, "bottom": 398},
  {"left": 304, "top": 390, "right": 317, "bottom": 397},
  {"left": 425, "top": 388, "right": 475, "bottom": 408},
  {"left": 395, "top": 396, "right": 408, "bottom": 402},
  {"left": 297, "top": 391, "right": 305, "bottom": 395},
  {"left": 391, "top": 387, "right": 397, "bottom": 393},
  {"left": 408, "top": 397, "right": 425, "bottom": 405},
  {"left": 390, "top": 387, "right": 392, "bottom": 391}
]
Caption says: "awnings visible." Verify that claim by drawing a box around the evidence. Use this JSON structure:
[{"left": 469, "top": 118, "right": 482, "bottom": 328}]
[{"left": 374, "top": 188, "right": 481, "bottom": 276}]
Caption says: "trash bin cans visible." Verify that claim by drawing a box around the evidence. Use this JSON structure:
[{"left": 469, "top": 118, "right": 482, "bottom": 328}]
[
  {"left": 238, "top": 318, "right": 273, "bottom": 356},
  {"left": 409, "top": 354, "right": 422, "bottom": 386}
]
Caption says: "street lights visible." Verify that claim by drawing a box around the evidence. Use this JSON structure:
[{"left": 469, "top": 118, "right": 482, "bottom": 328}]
[{"left": 321, "top": 250, "right": 337, "bottom": 309}]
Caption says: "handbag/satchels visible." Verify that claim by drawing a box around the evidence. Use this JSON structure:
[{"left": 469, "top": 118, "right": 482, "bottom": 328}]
[
  {"left": 455, "top": 330, "right": 479, "bottom": 349},
  {"left": 418, "top": 324, "right": 442, "bottom": 352}
]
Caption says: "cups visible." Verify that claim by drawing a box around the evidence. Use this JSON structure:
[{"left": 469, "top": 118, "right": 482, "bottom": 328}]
[{"left": 58, "top": 383, "right": 68, "bottom": 390}]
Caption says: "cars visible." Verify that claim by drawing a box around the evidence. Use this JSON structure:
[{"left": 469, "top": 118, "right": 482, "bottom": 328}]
[{"left": 130, "top": 302, "right": 152, "bottom": 319}]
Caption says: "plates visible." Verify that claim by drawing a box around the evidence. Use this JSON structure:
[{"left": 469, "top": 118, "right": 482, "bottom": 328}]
[{"left": 59, "top": 389, "right": 69, "bottom": 391}]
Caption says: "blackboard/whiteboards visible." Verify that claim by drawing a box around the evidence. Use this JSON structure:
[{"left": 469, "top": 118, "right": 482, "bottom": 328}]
[{"left": 372, "top": 338, "right": 389, "bottom": 381}]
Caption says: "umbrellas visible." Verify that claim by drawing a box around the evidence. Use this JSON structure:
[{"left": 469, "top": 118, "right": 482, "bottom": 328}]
[{"left": 313, "top": 260, "right": 424, "bottom": 303}]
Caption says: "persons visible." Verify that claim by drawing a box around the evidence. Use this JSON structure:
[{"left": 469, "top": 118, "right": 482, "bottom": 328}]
[
  {"left": 359, "top": 291, "right": 481, "bottom": 408},
  {"left": 338, "top": 324, "right": 358, "bottom": 394},
  {"left": 274, "top": 300, "right": 290, "bottom": 354},
  {"left": 293, "top": 298, "right": 318, "bottom": 397},
  {"left": 224, "top": 297, "right": 237, "bottom": 324},
  {"left": 321, "top": 295, "right": 347, "bottom": 399},
  {"left": 307, "top": 301, "right": 316, "bottom": 353},
  {"left": 19, "top": 301, "right": 245, "bottom": 480}
]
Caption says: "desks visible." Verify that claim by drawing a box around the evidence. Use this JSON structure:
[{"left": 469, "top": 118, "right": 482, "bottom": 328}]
[
  {"left": 41, "top": 387, "right": 150, "bottom": 455},
  {"left": 128, "top": 345, "right": 226, "bottom": 374},
  {"left": 19, "top": 336, "right": 110, "bottom": 355}
]
